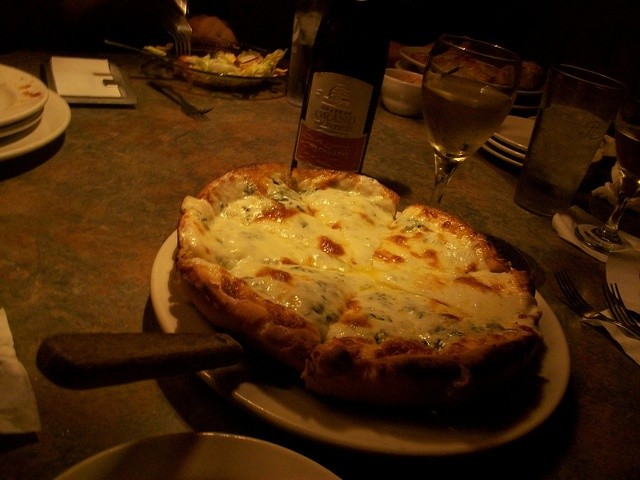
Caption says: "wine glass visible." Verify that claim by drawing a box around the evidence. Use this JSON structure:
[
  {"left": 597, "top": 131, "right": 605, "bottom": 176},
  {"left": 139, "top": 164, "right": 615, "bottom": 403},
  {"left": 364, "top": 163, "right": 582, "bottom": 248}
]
[
  {"left": 424, "top": 32, "right": 517, "bottom": 210},
  {"left": 574, "top": 96, "right": 640, "bottom": 257}
]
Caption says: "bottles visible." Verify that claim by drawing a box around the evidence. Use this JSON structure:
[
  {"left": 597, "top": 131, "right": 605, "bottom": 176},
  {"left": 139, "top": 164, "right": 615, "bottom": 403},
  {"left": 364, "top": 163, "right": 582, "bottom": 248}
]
[{"left": 290, "top": 0, "right": 390, "bottom": 173}]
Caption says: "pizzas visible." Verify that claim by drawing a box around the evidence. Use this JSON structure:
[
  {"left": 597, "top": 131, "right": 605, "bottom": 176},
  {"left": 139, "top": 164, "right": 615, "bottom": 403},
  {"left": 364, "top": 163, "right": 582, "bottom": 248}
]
[
  {"left": 409, "top": 52, "right": 498, "bottom": 88},
  {"left": 172, "top": 160, "right": 548, "bottom": 407}
]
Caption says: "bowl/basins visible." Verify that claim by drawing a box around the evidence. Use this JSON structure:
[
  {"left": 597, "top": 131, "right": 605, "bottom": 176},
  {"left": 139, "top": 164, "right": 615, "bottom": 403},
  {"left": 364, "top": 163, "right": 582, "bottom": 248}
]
[{"left": 379, "top": 68, "right": 424, "bottom": 117}]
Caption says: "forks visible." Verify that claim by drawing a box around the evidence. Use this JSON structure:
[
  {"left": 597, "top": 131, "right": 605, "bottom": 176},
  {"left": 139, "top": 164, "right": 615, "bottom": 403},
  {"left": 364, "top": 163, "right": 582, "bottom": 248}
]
[
  {"left": 167, "top": 0, "right": 194, "bottom": 56},
  {"left": 601, "top": 282, "right": 640, "bottom": 336},
  {"left": 554, "top": 268, "right": 640, "bottom": 340},
  {"left": 145, "top": 72, "right": 214, "bottom": 116}
]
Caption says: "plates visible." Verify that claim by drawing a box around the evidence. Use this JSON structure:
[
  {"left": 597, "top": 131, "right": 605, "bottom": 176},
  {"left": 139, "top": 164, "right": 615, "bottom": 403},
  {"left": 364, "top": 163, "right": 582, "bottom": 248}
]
[
  {"left": 141, "top": 36, "right": 288, "bottom": 92},
  {"left": 481, "top": 143, "right": 524, "bottom": 169},
  {"left": 487, "top": 137, "right": 527, "bottom": 161},
  {"left": 0, "top": 63, "right": 50, "bottom": 128},
  {"left": 52, "top": 431, "right": 344, "bottom": 479},
  {"left": 0, "top": 87, "right": 72, "bottom": 162},
  {"left": 492, "top": 114, "right": 604, "bottom": 167},
  {"left": 0, "top": 104, "right": 45, "bottom": 139},
  {"left": 394, "top": 58, "right": 542, "bottom": 114},
  {"left": 399, "top": 45, "right": 543, "bottom": 97},
  {"left": 149, "top": 224, "right": 573, "bottom": 457}
]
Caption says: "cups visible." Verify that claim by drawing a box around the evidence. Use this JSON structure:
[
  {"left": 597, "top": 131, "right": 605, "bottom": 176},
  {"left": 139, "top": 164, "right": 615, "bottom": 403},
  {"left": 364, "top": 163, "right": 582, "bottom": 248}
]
[
  {"left": 286, "top": 0, "right": 324, "bottom": 108},
  {"left": 513, "top": 62, "right": 629, "bottom": 221}
]
